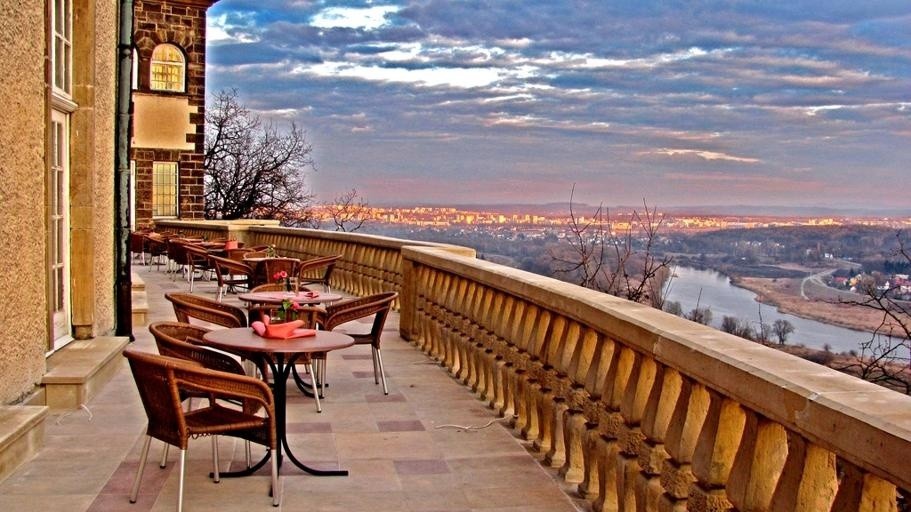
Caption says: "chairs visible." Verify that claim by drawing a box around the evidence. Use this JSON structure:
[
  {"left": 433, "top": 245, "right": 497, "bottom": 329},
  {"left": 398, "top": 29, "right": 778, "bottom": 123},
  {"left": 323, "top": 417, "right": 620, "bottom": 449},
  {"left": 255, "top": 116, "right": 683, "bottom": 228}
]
[
  {"left": 249, "top": 284, "right": 314, "bottom": 375},
  {"left": 164, "top": 291, "right": 247, "bottom": 373},
  {"left": 148, "top": 319, "right": 247, "bottom": 416},
  {"left": 316, "top": 291, "right": 399, "bottom": 399},
  {"left": 124, "top": 228, "right": 345, "bottom": 308},
  {"left": 122, "top": 347, "right": 280, "bottom": 508}
]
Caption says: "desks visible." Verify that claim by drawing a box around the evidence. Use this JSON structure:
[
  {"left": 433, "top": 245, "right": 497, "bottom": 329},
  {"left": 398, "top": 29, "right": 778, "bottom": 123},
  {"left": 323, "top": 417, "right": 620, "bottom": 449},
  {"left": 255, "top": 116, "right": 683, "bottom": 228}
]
[
  {"left": 203, "top": 328, "right": 355, "bottom": 507},
  {"left": 238, "top": 291, "right": 344, "bottom": 399}
]
